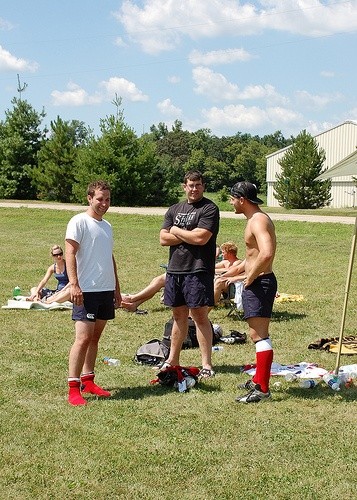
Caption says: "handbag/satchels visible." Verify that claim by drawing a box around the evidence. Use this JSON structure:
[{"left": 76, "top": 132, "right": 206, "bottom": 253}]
[
  {"left": 131, "top": 338, "right": 170, "bottom": 366},
  {"left": 163, "top": 316, "right": 215, "bottom": 350}
]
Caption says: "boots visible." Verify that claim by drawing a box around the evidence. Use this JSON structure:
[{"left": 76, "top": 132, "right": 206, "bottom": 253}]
[
  {"left": 81, "top": 374, "right": 111, "bottom": 397},
  {"left": 69, "top": 381, "right": 86, "bottom": 407}
]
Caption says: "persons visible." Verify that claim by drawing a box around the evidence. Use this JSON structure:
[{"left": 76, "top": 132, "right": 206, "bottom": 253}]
[
  {"left": 65, "top": 182, "right": 122, "bottom": 406},
  {"left": 150, "top": 172, "right": 219, "bottom": 383},
  {"left": 119, "top": 272, "right": 166, "bottom": 312},
  {"left": 26, "top": 245, "right": 74, "bottom": 303},
  {"left": 214, "top": 240, "right": 246, "bottom": 305},
  {"left": 229, "top": 181, "right": 277, "bottom": 404}
]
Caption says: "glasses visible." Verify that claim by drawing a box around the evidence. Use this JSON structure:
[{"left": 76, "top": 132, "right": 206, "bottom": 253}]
[{"left": 53, "top": 252, "right": 63, "bottom": 256}]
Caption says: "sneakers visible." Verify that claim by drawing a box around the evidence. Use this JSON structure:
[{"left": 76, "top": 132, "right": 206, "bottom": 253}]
[
  {"left": 219, "top": 329, "right": 247, "bottom": 344},
  {"left": 237, "top": 375, "right": 256, "bottom": 391},
  {"left": 235, "top": 383, "right": 270, "bottom": 404}
]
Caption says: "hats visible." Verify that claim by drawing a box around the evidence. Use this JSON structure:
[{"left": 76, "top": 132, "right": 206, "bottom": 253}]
[{"left": 231, "top": 181, "right": 264, "bottom": 205}]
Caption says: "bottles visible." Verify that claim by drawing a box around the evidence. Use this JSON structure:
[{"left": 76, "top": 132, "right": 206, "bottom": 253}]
[
  {"left": 285, "top": 373, "right": 300, "bottom": 382},
  {"left": 212, "top": 346, "right": 223, "bottom": 351},
  {"left": 323, "top": 374, "right": 341, "bottom": 391},
  {"left": 103, "top": 357, "right": 121, "bottom": 367},
  {"left": 13, "top": 286, "right": 21, "bottom": 298},
  {"left": 299, "top": 380, "right": 318, "bottom": 388}
]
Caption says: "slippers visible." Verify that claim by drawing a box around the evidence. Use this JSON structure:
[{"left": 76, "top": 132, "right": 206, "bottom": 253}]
[
  {"left": 197, "top": 369, "right": 217, "bottom": 378},
  {"left": 152, "top": 361, "right": 173, "bottom": 370}
]
